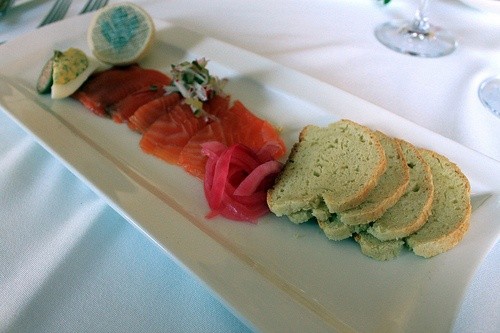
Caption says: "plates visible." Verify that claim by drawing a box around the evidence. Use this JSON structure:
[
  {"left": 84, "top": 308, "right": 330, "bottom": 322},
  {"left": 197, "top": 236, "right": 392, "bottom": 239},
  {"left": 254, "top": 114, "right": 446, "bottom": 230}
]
[{"left": 0, "top": 6, "right": 500, "bottom": 333}]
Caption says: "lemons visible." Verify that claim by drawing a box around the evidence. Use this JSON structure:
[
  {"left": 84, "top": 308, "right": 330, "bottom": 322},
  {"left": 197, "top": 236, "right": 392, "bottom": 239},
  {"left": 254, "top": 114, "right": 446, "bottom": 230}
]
[{"left": 87, "top": 2, "right": 156, "bottom": 65}]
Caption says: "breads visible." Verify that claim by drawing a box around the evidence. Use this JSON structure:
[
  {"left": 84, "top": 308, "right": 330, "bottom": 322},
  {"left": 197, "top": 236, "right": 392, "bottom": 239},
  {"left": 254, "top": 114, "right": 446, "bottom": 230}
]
[{"left": 265, "top": 117, "right": 472, "bottom": 263}]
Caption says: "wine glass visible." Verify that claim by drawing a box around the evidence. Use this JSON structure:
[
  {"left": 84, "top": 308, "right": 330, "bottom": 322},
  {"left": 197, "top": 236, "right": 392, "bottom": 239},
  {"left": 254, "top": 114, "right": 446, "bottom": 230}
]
[
  {"left": 374, "top": 0, "right": 457, "bottom": 57},
  {"left": 478, "top": 75, "right": 500, "bottom": 119}
]
